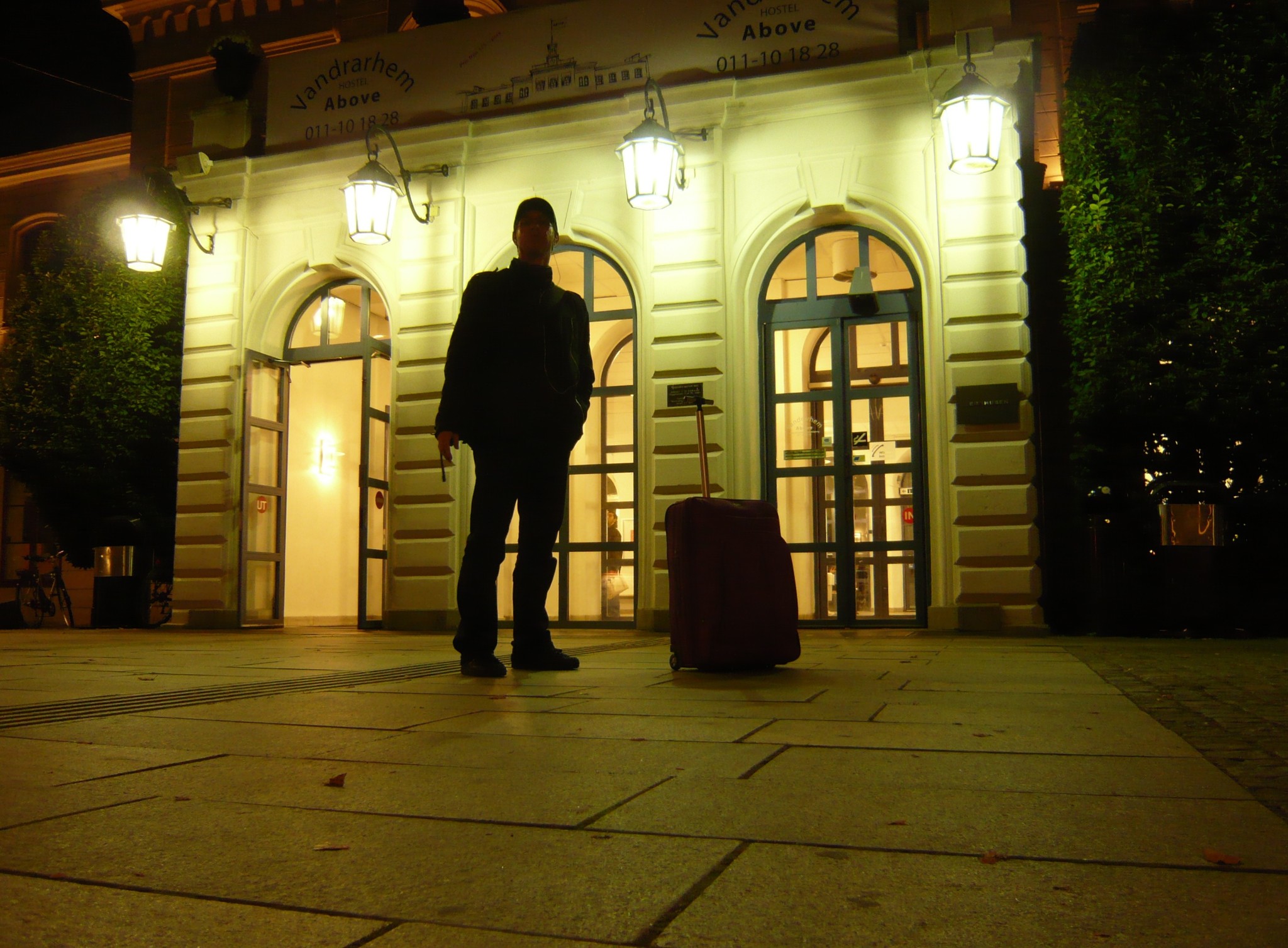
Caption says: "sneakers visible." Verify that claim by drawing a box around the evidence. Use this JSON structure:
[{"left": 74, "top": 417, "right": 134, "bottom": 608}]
[
  {"left": 461, "top": 653, "right": 507, "bottom": 678},
  {"left": 510, "top": 644, "right": 580, "bottom": 670}
]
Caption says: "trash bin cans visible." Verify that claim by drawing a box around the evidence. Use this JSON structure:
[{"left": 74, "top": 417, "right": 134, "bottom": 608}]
[
  {"left": 1149, "top": 482, "right": 1231, "bottom": 636},
  {"left": 90, "top": 515, "right": 154, "bottom": 628}
]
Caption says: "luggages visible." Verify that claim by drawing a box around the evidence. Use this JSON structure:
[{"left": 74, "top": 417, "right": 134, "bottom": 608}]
[{"left": 664, "top": 400, "right": 800, "bottom": 676}]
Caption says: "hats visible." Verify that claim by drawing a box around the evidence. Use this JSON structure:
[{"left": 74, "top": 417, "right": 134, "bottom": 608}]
[{"left": 514, "top": 198, "right": 558, "bottom": 234}]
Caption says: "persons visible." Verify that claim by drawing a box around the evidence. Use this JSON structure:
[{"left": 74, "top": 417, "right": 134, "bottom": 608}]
[{"left": 432, "top": 197, "right": 596, "bottom": 678}]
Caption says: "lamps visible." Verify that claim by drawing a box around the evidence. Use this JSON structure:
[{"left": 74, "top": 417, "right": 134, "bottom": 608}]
[
  {"left": 615, "top": 77, "right": 686, "bottom": 210},
  {"left": 114, "top": 164, "right": 218, "bottom": 273},
  {"left": 338, "top": 124, "right": 431, "bottom": 247},
  {"left": 933, "top": 30, "right": 1011, "bottom": 175}
]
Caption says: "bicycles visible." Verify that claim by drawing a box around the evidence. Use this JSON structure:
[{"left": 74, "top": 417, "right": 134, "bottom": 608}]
[{"left": 13, "top": 549, "right": 75, "bottom": 630}]
